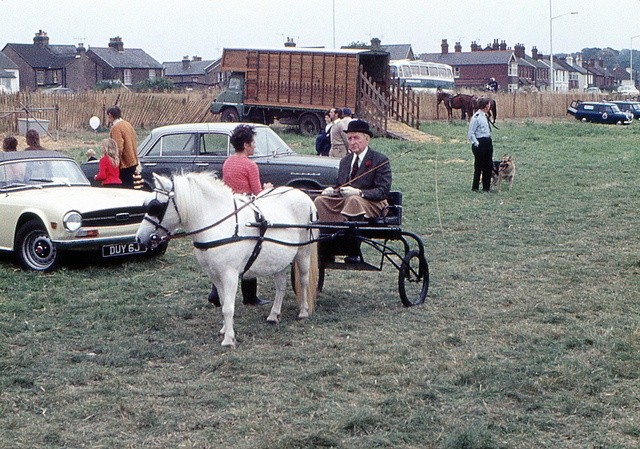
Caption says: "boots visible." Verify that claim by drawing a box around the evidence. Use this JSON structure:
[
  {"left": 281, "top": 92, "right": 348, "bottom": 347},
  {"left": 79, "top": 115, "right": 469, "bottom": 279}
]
[
  {"left": 208, "top": 288, "right": 221, "bottom": 308},
  {"left": 242, "top": 278, "right": 270, "bottom": 307}
]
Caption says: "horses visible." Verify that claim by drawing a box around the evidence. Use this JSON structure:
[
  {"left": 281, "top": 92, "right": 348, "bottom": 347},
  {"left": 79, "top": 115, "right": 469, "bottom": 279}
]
[
  {"left": 135, "top": 168, "right": 320, "bottom": 348},
  {"left": 437, "top": 92, "right": 471, "bottom": 119},
  {"left": 469, "top": 94, "right": 496, "bottom": 123}
]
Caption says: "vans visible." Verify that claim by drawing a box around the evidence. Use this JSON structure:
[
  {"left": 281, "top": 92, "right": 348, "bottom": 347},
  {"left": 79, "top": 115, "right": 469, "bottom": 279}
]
[{"left": 570, "top": 100, "right": 633, "bottom": 125}]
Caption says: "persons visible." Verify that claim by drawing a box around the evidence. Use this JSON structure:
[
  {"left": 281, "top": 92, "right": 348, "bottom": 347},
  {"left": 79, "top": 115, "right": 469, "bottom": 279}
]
[
  {"left": 313, "top": 107, "right": 355, "bottom": 157},
  {"left": 107, "top": 106, "right": 139, "bottom": 188},
  {"left": 201, "top": 124, "right": 273, "bottom": 306},
  {"left": 467, "top": 98, "right": 492, "bottom": 192},
  {"left": 2, "top": 137, "right": 19, "bottom": 152},
  {"left": 25, "top": 129, "right": 45, "bottom": 151},
  {"left": 94, "top": 138, "right": 122, "bottom": 188},
  {"left": 314, "top": 121, "right": 391, "bottom": 234},
  {"left": 85, "top": 150, "right": 97, "bottom": 161},
  {"left": 133, "top": 163, "right": 146, "bottom": 189}
]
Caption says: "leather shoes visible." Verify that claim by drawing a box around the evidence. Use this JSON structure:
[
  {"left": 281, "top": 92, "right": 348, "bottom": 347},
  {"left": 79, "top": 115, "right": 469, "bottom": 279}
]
[{"left": 343, "top": 256, "right": 358, "bottom": 264}]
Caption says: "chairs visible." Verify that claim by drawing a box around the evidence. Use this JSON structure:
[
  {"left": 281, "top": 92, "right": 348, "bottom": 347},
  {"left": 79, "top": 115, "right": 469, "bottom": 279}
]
[{"left": 326, "top": 192, "right": 402, "bottom": 223}]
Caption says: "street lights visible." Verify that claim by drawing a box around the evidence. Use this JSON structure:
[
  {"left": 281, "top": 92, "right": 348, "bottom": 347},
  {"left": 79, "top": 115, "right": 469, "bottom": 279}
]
[{"left": 549, "top": 0, "right": 579, "bottom": 91}]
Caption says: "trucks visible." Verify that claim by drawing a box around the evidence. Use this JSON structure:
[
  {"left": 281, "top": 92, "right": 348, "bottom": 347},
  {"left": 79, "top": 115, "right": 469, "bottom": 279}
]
[{"left": 204, "top": 45, "right": 443, "bottom": 145}]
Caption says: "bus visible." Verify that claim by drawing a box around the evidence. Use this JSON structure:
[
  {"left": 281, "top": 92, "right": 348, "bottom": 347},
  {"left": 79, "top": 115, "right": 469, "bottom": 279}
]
[{"left": 389, "top": 57, "right": 456, "bottom": 98}]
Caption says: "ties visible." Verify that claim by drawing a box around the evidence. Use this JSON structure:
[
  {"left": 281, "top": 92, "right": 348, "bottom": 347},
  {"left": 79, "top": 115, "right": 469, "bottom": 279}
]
[
  {"left": 485, "top": 113, "right": 500, "bottom": 133},
  {"left": 350, "top": 155, "right": 359, "bottom": 180}
]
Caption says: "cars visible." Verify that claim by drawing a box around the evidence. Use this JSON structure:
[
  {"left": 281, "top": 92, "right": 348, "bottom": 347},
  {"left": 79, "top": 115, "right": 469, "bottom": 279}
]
[
  {"left": 616, "top": 101, "right": 640, "bottom": 121},
  {"left": 82, "top": 123, "right": 343, "bottom": 200},
  {"left": 0, "top": 151, "right": 168, "bottom": 273}
]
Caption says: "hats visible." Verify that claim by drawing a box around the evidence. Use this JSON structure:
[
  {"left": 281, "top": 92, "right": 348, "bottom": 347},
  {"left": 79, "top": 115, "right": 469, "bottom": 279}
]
[
  {"left": 342, "top": 107, "right": 352, "bottom": 115},
  {"left": 342, "top": 120, "right": 373, "bottom": 138}
]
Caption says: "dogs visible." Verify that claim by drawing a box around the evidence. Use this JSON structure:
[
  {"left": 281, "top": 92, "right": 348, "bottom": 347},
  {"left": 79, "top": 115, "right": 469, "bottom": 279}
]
[
  {"left": 498, "top": 154, "right": 514, "bottom": 193},
  {"left": 479, "top": 160, "right": 502, "bottom": 188}
]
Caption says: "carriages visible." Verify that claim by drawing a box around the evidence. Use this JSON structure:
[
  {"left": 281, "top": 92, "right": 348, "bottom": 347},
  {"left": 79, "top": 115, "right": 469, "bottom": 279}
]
[{"left": 136, "top": 171, "right": 429, "bottom": 346}]
